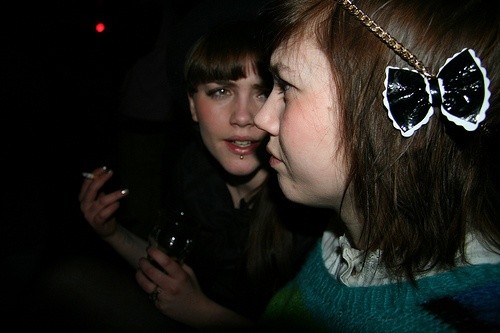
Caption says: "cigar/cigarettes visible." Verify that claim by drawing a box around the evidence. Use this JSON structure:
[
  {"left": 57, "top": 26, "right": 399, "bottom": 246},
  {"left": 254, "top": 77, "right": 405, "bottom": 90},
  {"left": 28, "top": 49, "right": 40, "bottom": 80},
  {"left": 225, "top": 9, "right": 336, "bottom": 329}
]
[{"left": 80, "top": 170, "right": 98, "bottom": 181}]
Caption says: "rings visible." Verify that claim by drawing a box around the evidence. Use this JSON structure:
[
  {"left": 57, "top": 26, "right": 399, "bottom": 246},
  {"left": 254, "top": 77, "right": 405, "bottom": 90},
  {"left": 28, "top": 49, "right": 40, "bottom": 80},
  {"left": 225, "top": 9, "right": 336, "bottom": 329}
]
[{"left": 145, "top": 284, "right": 161, "bottom": 303}]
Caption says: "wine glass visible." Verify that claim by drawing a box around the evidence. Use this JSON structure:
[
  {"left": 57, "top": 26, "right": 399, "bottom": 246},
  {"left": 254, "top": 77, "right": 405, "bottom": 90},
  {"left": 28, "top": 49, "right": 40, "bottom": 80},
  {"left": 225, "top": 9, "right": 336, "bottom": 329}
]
[{"left": 137, "top": 208, "right": 203, "bottom": 320}]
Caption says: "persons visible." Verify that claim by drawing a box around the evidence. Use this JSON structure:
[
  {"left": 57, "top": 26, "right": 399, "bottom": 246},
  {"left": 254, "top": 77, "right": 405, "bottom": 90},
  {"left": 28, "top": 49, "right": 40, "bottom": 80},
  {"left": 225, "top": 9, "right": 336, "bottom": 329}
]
[
  {"left": 242, "top": 0, "right": 500, "bottom": 333},
  {"left": 73, "top": 14, "right": 310, "bottom": 333}
]
